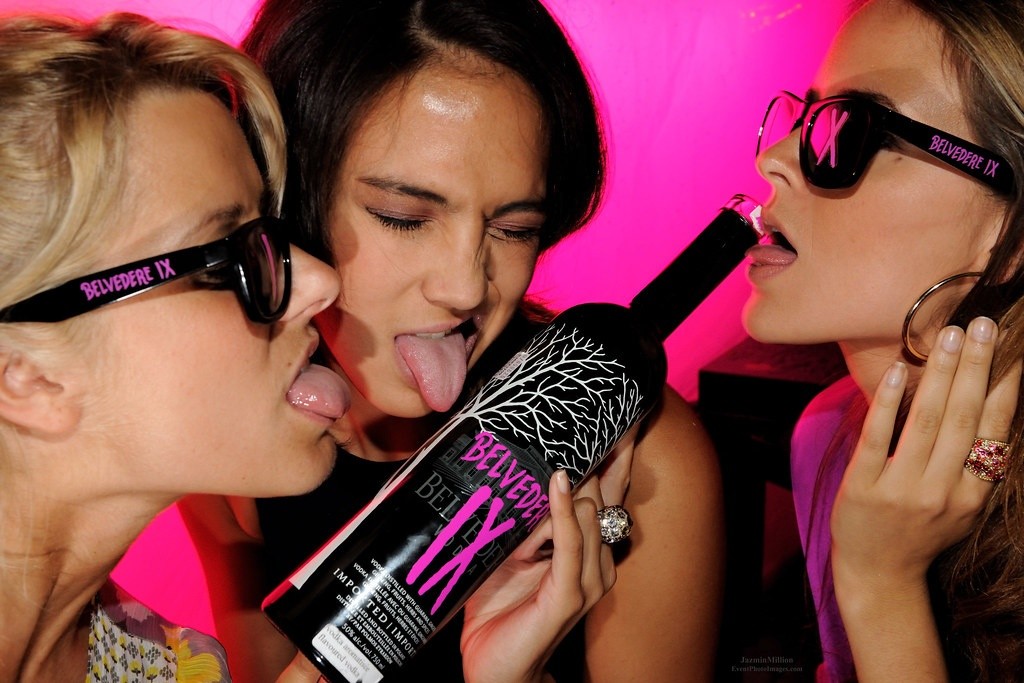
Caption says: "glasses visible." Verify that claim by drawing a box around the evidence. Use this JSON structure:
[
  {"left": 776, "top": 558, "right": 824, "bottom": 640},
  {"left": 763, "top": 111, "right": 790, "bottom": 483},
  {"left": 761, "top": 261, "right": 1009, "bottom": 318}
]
[
  {"left": 756, "top": 89, "right": 1020, "bottom": 204},
  {"left": 0, "top": 203, "right": 329, "bottom": 324}
]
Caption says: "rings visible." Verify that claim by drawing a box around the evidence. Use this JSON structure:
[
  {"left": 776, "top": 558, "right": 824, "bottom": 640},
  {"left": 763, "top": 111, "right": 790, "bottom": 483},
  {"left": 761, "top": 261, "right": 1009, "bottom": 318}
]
[
  {"left": 597, "top": 504, "right": 633, "bottom": 542},
  {"left": 964, "top": 438, "right": 1014, "bottom": 483}
]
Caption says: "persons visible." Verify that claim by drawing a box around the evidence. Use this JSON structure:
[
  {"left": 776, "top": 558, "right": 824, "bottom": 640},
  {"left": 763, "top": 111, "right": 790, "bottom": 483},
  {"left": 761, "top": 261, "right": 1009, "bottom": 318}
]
[
  {"left": 743, "top": 0, "right": 1024, "bottom": 683},
  {"left": 177, "top": 0, "right": 726, "bottom": 682},
  {"left": 0, "top": 13, "right": 351, "bottom": 683}
]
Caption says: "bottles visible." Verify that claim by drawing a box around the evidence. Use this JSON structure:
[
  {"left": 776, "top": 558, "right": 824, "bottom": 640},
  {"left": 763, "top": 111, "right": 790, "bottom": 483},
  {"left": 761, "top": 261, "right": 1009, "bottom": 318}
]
[{"left": 260, "top": 188, "right": 777, "bottom": 683}]
